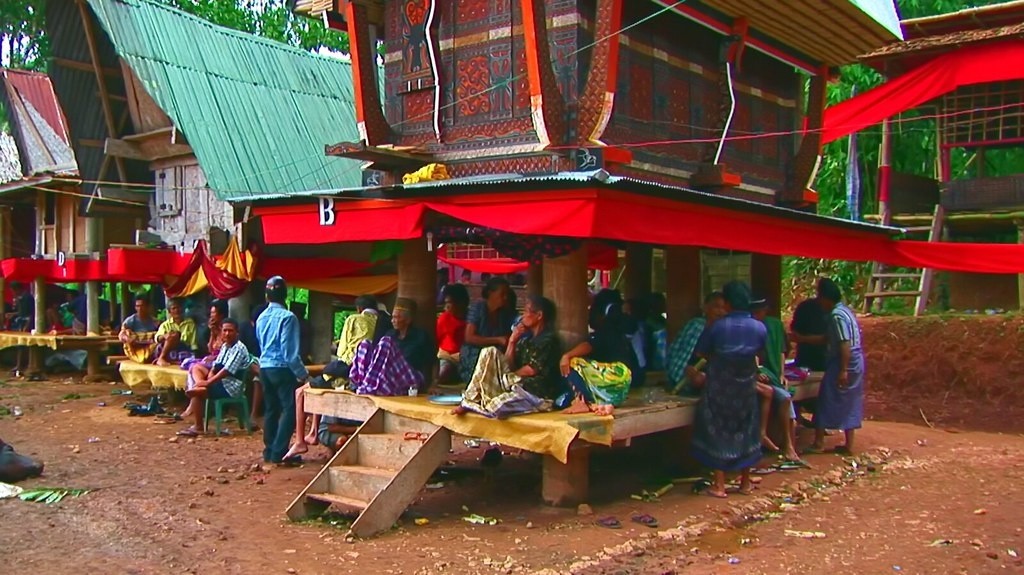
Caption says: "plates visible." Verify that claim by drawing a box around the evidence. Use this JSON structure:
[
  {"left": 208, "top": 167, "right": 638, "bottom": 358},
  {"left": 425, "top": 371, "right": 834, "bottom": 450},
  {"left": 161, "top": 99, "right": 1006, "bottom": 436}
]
[{"left": 428, "top": 395, "right": 463, "bottom": 405}]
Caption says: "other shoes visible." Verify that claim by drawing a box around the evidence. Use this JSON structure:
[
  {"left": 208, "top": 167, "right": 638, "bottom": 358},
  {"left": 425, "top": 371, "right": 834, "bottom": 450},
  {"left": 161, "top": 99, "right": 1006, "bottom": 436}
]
[
  {"left": 287, "top": 454, "right": 302, "bottom": 461},
  {"left": 176, "top": 428, "right": 198, "bottom": 436}
]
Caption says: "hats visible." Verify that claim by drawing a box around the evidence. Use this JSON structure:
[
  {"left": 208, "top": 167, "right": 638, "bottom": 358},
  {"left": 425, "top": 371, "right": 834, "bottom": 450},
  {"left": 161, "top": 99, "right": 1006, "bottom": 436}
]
[
  {"left": 266, "top": 274, "right": 287, "bottom": 295},
  {"left": 393, "top": 298, "right": 417, "bottom": 312}
]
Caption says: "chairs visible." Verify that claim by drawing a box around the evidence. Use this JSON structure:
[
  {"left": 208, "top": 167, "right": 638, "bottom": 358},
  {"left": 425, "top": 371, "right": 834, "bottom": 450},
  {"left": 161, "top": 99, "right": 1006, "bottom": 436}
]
[{"left": 205, "top": 353, "right": 254, "bottom": 436}]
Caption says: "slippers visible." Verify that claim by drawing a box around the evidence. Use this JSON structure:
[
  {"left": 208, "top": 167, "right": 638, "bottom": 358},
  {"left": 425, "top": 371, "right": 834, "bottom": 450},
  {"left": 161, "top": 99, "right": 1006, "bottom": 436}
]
[
  {"left": 633, "top": 513, "right": 659, "bottom": 527},
  {"left": 598, "top": 515, "right": 622, "bottom": 529}
]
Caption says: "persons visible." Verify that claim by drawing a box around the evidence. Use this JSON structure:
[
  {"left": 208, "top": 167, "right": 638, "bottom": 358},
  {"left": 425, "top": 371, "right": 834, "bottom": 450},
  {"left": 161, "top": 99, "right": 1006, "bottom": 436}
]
[
  {"left": 68, "top": 282, "right": 110, "bottom": 336},
  {"left": 350, "top": 297, "right": 430, "bottom": 395},
  {"left": 438, "top": 276, "right": 518, "bottom": 385},
  {"left": 749, "top": 295, "right": 799, "bottom": 462},
  {"left": 118, "top": 295, "right": 161, "bottom": 364},
  {"left": 450, "top": 297, "right": 562, "bottom": 415},
  {"left": 6, "top": 281, "right": 34, "bottom": 331},
  {"left": 787, "top": 278, "right": 865, "bottom": 454},
  {"left": 790, "top": 296, "right": 835, "bottom": 437},
  {"left": 669, "top": 291, "right": 728, "bottom": 391},
  {"left": 248, "top": 274, "right": 313, "bottom": 465},
  {"left": 559, "top": 287, "right": 667, "bottom": 417},
  {"left": 153, "top": 297, "right": 197, "bottom": 366},
  {"left": 177, "top": 318, "right": 250, "bottom": 436},
  {"left": 694, "top": 278, "right": 766, "bottom": 497},
  {"left": 180, "top": 298, "right": 228, "bottom": 418},
  {"left": 281, "top": 294, "right": 389, "bottom": 461}
]
